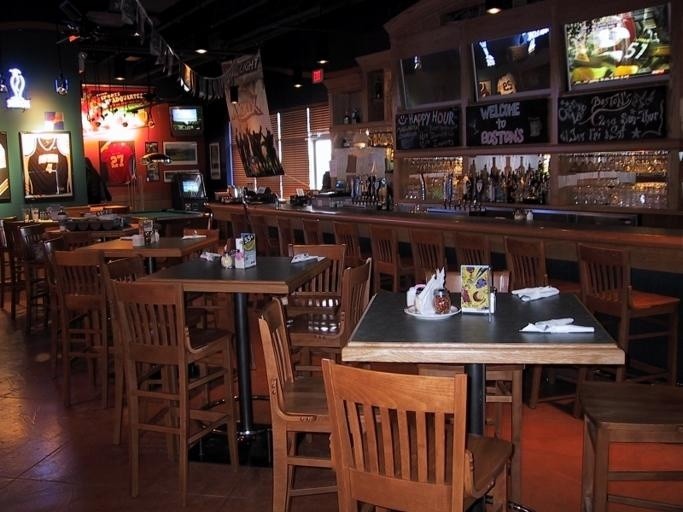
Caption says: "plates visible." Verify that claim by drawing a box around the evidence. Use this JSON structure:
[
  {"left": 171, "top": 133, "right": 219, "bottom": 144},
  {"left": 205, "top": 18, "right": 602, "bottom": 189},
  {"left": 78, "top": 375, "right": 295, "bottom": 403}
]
[{"left": 403, "top": 303, "right": 462, "bottom": 322}]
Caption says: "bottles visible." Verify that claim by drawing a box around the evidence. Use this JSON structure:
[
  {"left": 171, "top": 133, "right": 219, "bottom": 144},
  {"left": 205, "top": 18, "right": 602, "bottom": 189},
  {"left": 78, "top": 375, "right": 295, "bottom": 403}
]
[
  {"left": 514, "top": 209, "right": 525, "bottom": 222},
  {"left": 463, "top": 156, "right": 549, "bottom": 203},
  {"left": 221, "top": 252, "right": 233, "bottom": 269},
  {"left": 526, "top": 209, "right": 533, "bottom": 221},
  {"left": 226, "top": 184, "right": 246, "bottom": 203},
  {"left": 343, "top": 108, "right": 360, "bottom": 125},
  {"left": 368, "top": 130, "right": 393, "bottom": 147},
  {"left": 375, "top": 83, "right": 381, "bottom": 99},
  {"left": 58, "top": 215, "right": 66, "bottom": 232},
  {"left": 433, "top": 288, "right": 451, "bottom": 314},
  {"left": 24, "top": 208, "right": 65, "bottom": 222},
  {"left": 405, "top": 173, "right": 463, "bottom": 200},
  {"left": 66, "top": 217, "right": 120, "bottom": 231}
]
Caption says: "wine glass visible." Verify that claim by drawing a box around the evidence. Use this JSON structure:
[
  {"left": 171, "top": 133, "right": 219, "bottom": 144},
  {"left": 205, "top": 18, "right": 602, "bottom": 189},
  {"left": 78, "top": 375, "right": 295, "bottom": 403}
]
[{"left": 559, "top": 150, "right": 669, "bottom": 174}]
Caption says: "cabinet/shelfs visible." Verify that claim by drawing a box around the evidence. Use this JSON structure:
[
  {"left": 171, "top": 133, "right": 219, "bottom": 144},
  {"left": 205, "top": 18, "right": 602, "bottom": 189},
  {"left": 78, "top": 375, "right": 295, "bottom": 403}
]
[{"left": 398, "top": 148, "right": 669, "bottom": 213}]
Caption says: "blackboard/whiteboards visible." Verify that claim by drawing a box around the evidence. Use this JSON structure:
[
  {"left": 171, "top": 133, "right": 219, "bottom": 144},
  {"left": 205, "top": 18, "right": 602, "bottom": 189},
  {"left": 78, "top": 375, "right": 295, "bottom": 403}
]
[
  {"left": 466, "top": 99, "right": 548, "bottom": 146},
  {"left": 396, "top": 109, "right": 460, "bottom": 149},
  {"left": 557, "top": 85, "right": 666, "bottom": 143}
]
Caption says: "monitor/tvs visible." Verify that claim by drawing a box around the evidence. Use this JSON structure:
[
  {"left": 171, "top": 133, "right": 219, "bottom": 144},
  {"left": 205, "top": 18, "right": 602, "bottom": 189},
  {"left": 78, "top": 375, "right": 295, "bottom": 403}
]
[{"left": 176, "top": 172, "right": 208, "bottom": 203}]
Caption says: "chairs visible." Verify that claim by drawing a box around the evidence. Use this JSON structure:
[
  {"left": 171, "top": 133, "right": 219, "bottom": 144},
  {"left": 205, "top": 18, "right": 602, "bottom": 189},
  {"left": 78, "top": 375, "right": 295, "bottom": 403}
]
[
  {"left": 580, "top": 374, "right": 683, "bottom": 511},
  {"left": 577, "top": 238, "right": 679, "bottom": 384}
]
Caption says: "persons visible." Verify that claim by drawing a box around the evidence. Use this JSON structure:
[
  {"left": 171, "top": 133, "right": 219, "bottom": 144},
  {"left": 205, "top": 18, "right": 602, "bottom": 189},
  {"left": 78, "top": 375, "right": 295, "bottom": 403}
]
[{"left": 236, "top": 124, "right": 284, "bottom": 178}]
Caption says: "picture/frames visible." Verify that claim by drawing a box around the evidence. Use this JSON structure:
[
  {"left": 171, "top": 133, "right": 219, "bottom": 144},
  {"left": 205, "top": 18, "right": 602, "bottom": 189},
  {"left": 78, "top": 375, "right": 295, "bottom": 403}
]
[
  {"left": 18, "top": 130, "right": 75, "bottom": 202},
  {"left": 163, "top": 141, "right": 198, "bottom": 165}
]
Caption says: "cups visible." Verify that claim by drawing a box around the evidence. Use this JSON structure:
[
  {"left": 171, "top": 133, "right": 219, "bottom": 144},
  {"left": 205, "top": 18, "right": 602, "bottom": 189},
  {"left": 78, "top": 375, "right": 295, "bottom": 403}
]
[
  {"left": 132, "top": 235, "right": 144, "bottom": 247},
  {"left": 409, "top": 157, "right": 463, "bottom": 173},
  {"left": 576, "top": 176, "right": 668, "bottom": 210}
]
